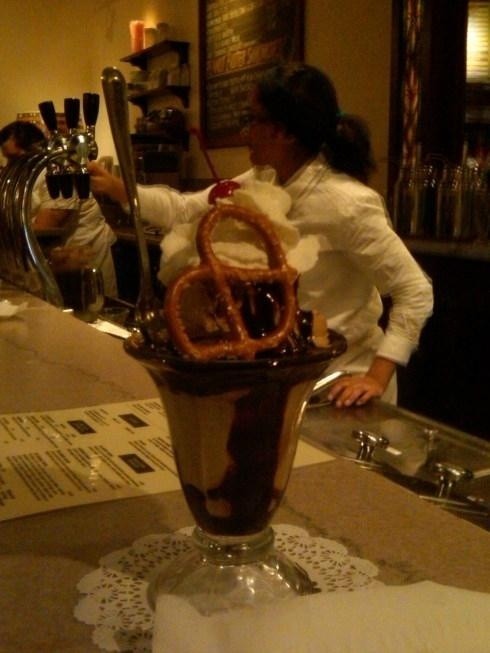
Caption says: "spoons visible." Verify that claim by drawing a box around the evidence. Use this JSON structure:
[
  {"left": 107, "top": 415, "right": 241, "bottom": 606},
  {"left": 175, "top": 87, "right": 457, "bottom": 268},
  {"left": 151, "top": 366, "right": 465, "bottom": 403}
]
[{"left": 100, "top": 65, "right": 169, "bottom": 352}]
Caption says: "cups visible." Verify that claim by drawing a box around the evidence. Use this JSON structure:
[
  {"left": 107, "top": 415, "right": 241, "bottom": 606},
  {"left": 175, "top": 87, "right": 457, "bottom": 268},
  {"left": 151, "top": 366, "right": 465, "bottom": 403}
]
[{"left": 127, "top": 20, "right": 169, "bottom": 55}]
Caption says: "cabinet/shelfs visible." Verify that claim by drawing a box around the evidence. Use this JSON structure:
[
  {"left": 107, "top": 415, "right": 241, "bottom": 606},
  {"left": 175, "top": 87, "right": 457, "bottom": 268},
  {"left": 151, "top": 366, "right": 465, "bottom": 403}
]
[{"left": 120, "top": 39, "right": 190, "bottom": 115}]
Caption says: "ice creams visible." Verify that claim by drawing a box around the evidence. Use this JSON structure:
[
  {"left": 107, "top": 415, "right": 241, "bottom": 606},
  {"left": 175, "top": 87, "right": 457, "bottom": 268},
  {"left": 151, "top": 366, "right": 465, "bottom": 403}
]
[{"left": 137, "top": 127, "right": 331, "bottom": 536}]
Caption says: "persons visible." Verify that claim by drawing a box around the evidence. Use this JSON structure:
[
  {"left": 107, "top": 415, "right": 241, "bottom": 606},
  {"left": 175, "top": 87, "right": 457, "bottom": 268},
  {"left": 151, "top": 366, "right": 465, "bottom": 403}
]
[
  {"left": 1, "top": 119, "right": 120, "bottom": 304},
  {"left": 82, "top": 63, "right": 432, "bottom": 409}
]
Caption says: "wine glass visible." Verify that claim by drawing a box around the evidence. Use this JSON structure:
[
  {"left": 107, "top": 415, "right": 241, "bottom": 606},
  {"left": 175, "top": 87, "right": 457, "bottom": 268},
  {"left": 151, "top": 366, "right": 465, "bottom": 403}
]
[{"left": 119, "top": 324, "right": 347, "bottom": 623}]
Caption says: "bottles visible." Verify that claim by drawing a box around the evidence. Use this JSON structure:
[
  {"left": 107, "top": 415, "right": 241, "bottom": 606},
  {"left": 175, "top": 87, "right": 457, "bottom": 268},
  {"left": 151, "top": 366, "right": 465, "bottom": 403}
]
[
  {"left": 147, "top": 62, "right": 189, "bottom": 88},
  {"left": 390, "top": 152, "right": 482, "bottom": 242},
  {"left": 48, "top": 243, "right": 106, "bottom": 314}
]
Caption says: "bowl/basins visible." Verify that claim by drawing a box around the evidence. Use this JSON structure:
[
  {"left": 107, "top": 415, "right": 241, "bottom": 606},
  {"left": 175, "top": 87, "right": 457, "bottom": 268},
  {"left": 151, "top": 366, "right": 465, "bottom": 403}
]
[{"left": 126, "top": 68, "right": 148, "bottom": 95}]
